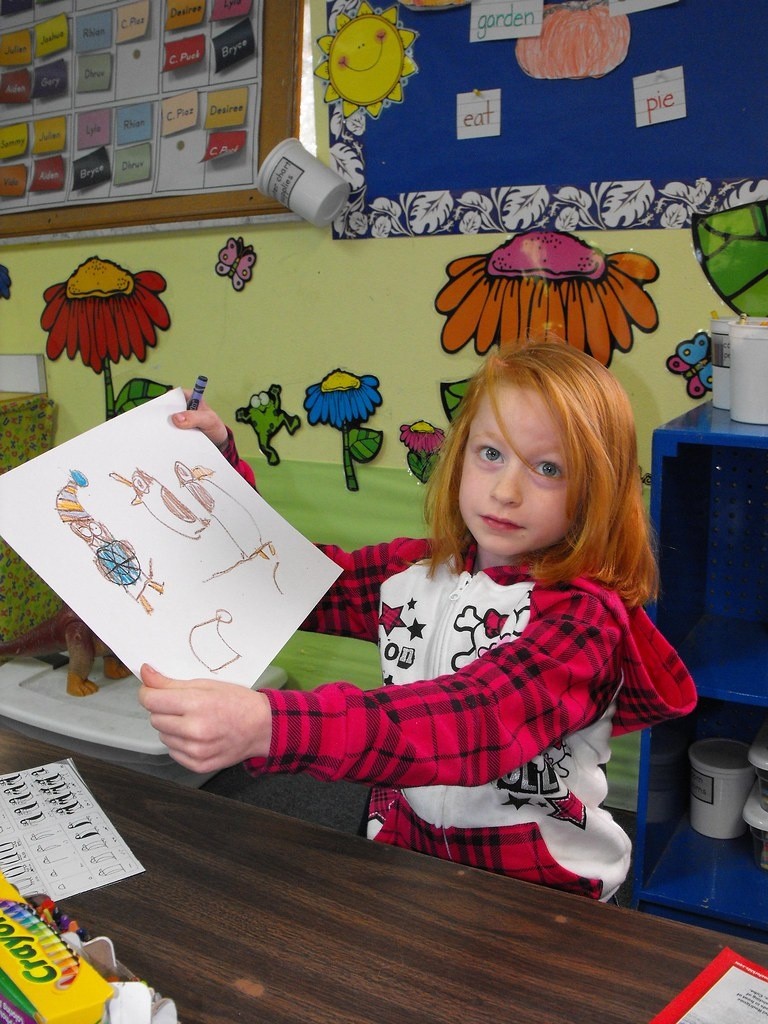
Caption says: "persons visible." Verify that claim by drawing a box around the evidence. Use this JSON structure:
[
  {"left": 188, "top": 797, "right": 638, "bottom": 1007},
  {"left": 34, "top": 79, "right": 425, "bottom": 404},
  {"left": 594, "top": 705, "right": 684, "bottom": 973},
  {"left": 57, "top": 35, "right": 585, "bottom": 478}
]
[{"left": 137, "top": 343, "right": 697, "bottom": 907}]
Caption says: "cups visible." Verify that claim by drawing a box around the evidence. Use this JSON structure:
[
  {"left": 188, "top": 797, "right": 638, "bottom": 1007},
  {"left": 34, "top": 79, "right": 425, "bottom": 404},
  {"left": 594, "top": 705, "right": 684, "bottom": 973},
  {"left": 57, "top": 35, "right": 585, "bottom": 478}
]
[
  {"left": 256, "top": 138, "right": 350, "bottom": 227},
  {"left": 727, "top": 321, "right": 768, "bottom": 425},
  {"left": 710, "top": 317, "right": 768, "bottom": 410},
  {"left": 688, "top": 739, "right": 756, "bottom": 840}
]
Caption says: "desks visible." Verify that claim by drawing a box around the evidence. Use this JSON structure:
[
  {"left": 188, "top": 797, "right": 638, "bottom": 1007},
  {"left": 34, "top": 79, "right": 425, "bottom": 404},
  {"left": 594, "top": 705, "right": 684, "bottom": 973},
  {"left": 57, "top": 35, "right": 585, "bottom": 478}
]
[{"left": 0, "top": 729, "right": 768, "bottom": 1024}]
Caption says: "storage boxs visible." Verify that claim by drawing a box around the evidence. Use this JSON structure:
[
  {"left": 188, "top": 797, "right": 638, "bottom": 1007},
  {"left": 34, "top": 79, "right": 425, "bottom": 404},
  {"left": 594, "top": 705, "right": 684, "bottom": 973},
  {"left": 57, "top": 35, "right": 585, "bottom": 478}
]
[{"left": 0, "top": 650, "right": 289, "bottom": 792}]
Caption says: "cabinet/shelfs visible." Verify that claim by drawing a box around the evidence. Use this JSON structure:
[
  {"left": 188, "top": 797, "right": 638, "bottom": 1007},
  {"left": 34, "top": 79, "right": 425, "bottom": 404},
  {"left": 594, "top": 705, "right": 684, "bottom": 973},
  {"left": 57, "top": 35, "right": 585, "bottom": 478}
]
[{"left": 628, "top": 401, "right": 768, "bottom": 944}]
